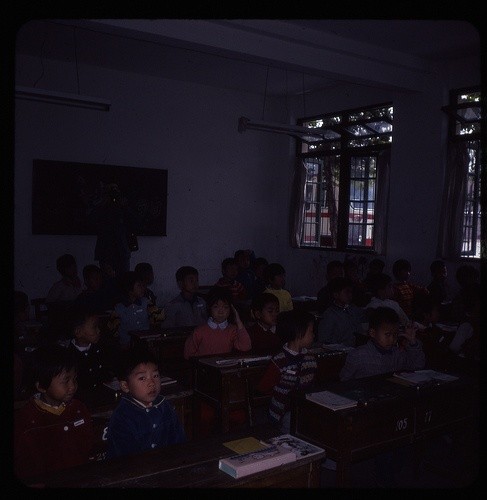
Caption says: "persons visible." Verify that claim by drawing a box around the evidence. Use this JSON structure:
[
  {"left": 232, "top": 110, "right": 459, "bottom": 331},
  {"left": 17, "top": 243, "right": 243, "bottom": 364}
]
[
  {"left": 391, "top": 259, "right": 430, "bottom": 319},
  {"left": 215, "top": 250, "right": 268, "bottom": 302},
  {"left": 261, "top": 263, "right": 293, "bottom": 313},
  {"left": 318, "top": 276, "right": 369, "bottom": 342},
  {"left": 340, "top": 306, "right": 422, "bottom": 382},
  {"left": 412, "top": 260, "right": 479, "bottom": 371},
  {"left": 106, "top": 354, "right": 185, "bottom": 454},
  {"left": 162, "top": 265, "right": 208, "bottom": 336},
  {"left": 267, "top": 313, "right": 321, "bottom": 436},
  {"left": 14, "top": 348, "right": 98, "bottom": 481},
  {"left": 316, "top": 259, "right": 384, "bottom": 312},
  {"left": 183, "top": 287, "right": 252, "bottom": 360},
  {"left": 365, "top": 273, "right": 409, "bottom": 336},
  {"left": 249, "top": 293, "right": 283, "bottom": 348},
  {"left": 14, "top": 255, "right": 157, "bottom": 394}
]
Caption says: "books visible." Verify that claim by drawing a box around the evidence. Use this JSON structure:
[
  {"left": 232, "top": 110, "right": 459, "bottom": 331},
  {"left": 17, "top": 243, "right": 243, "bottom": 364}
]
[
  {"left": 160, "top": 376, "right": 177, "bottom": 386},
  {"left": 305, "top": 390, "right": 359, "bottom": 412},
  {"left": 261, "top": 433, "right": 326, "bottom": 461}
]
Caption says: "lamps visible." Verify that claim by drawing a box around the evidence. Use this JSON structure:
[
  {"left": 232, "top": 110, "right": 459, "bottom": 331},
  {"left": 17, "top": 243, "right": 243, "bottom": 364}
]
[
  {"left": 238, "top": 63, "right": 312, "bottom": 139},
  {"left": 14, "top": 19, "right": 113, "bottom": 112}
]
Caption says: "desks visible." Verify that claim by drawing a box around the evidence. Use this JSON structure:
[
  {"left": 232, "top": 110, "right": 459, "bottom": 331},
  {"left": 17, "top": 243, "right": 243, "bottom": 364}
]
[{"left": 14, "top": 290, "right": 480, "bottom": 488}]
